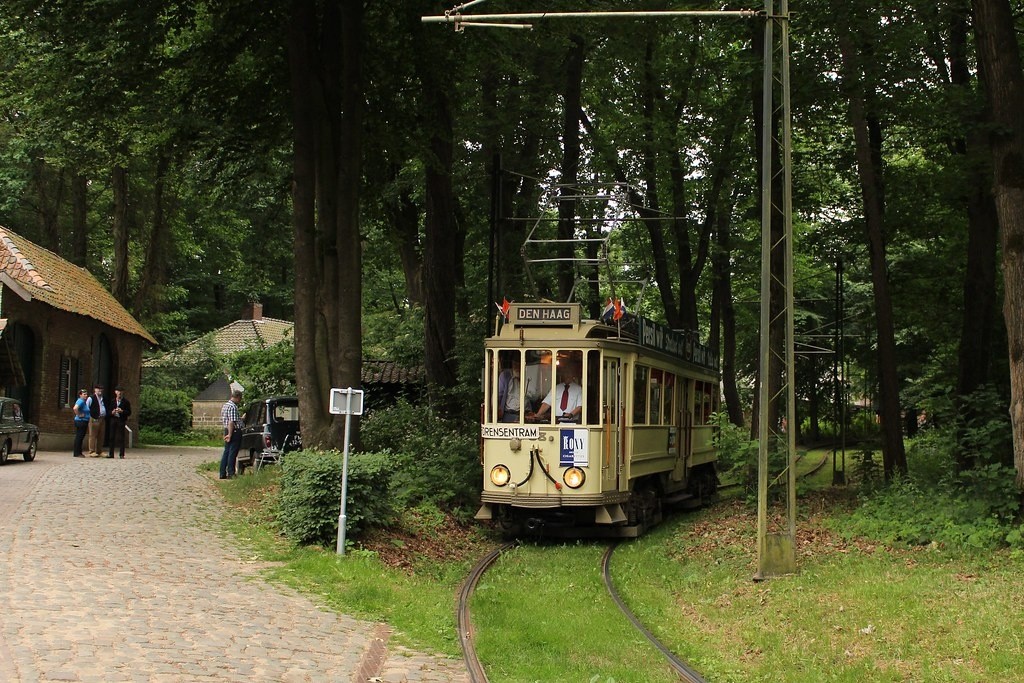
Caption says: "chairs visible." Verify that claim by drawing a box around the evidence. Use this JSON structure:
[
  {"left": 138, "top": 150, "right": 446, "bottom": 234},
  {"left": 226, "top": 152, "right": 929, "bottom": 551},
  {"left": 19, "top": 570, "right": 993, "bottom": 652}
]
[{"left": 255, "top": 434, "right": 290, "bottom": 476}]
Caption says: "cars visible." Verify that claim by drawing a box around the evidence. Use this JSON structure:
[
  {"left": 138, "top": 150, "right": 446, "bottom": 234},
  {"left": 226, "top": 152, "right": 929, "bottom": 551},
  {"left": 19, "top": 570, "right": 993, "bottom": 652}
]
[
  {"left": 237, "top": 396, "right": 302, "bottom": 474},
  {"left": 0, "top": 397, "right": 41, "bottom": 466}
]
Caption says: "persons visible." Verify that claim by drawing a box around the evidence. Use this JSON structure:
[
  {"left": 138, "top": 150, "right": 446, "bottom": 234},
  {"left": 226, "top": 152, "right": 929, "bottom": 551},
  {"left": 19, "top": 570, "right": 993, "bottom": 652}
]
[
  {"left": 219, "top": 390, "right": 245, "bottom": 479},
  {"left": 108, "top": 386, "right": 131, "bottom": 459},
  {"left": 73, "top": 389, "right": 90, "bottom": 458},
  {"left": 498, "top": 355, "right": 532, "bottom": 423},
  {"left": 531, "top": 366, "right": 582, "bottom": 425},
  {"left": 86, "top": 385, "right": 108, "bottom": 458}
]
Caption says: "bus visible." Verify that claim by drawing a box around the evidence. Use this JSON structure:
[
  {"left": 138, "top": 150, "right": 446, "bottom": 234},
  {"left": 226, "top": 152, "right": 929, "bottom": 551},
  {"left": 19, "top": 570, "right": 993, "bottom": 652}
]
[{"left": 472, "top": 298, "right": 722, "bottom": 540}]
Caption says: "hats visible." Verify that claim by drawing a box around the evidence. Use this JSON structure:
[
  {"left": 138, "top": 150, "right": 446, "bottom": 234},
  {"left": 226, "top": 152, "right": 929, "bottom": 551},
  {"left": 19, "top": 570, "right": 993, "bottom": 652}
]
[
  {"left": 77, "top": 389, "right": 87, "bottom": 395},
  {"left": 115, "top": 386, "right": 125, "bottom": 391},
  {"left": 94, "top": 384, "right": 104, "bottom": 389}
]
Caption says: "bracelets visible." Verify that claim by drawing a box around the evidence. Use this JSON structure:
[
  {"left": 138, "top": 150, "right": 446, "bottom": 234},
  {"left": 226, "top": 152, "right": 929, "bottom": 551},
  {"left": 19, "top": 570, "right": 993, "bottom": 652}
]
[{"left": 570, "top": 413, "right": 573, "bottom": 417}]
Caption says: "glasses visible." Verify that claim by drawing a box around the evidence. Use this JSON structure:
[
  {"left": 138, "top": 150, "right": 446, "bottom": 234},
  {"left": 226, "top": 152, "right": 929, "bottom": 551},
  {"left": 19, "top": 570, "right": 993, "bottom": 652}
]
[{"left": 81, "top": 389, "right": 87, "bottom": 392}]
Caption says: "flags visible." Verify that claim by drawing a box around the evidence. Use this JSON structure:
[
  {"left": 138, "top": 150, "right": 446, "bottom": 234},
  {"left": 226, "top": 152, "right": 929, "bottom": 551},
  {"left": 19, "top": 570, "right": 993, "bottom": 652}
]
[
  {"left": 602, "top": 296, "right": 628, "bottom": 322},
  {"left": 495, "top": 298, "right": 510, "bottom": 318}
]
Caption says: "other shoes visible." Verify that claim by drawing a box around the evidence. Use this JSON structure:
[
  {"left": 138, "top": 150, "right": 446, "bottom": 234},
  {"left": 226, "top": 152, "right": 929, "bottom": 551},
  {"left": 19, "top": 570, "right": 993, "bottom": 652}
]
[
  {"left": 106, "top": 456, "right": 114, "bottom": 458},
  {"left": 89, "top": 451, "right": 98, "bottom": 457},
  {"left": 98, "top": 454, "right": 107, "bottom": 458},
  {"left": 120, "top": 456, "right": 124, "bottom": 459},
  {"left": 220, "top": 475, "right": 238, "bottom": 479},
  {"left": 78, "top": 454, "right": 85, "bottom": 458}
]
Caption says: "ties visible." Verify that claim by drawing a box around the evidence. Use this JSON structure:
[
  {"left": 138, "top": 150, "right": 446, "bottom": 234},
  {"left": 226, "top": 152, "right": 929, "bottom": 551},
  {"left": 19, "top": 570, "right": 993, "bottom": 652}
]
[{"left": 560, "top": 385, "right": 570, "bottom": 411}]
